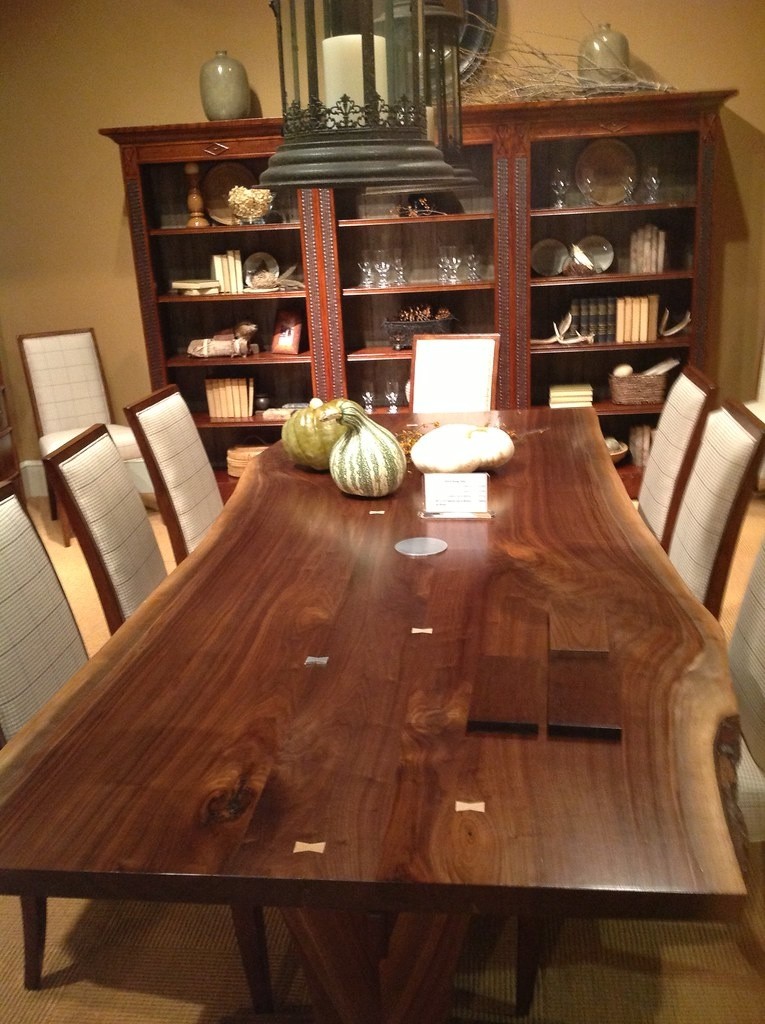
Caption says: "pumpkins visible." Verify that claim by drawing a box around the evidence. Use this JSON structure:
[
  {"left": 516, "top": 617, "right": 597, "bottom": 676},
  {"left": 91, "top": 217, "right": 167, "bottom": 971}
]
[
  {"left": 282, "top": 397, "right": 407, "bottom": 497},
  {"left": 410, "top": 424, "right": 514, "bottom": 474}
]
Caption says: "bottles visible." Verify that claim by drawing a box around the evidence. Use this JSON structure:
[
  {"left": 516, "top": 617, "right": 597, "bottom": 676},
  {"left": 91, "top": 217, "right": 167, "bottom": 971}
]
[
  {"left": 579, "top": 23, "right": 628, "bottom": 96},
  {"left": 200, "top": 50, "right": 250, "bottom": 120}
]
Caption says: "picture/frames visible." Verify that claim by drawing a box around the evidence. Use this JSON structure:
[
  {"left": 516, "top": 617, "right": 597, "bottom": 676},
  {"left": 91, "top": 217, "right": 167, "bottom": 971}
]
[{"left": 271, "top": 310, "right": 303, "bottom": 355}]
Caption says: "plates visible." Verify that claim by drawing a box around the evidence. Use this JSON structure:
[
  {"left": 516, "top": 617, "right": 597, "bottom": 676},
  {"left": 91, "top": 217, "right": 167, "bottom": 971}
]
[
  {"left": 531, "top": 239, "right": 569, "bottom": 276},
  {"left": 205, "top": 163, "right": 257, "bottom": 225},
  {"left": 244, "top": 253, "right": 280, "bottom": 289},
  {"left": 575, "top": 139, "right": 638, "bottom": 204},
  {"left": 573, "top": 236, "right": 614, "bottom": 274}
]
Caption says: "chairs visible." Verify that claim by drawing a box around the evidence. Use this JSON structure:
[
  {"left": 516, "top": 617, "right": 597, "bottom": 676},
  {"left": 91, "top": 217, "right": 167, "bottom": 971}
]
[
  {"left": 408, "top": 333, "right": 503, "bottom": 413},
  {"left": 514, "top": 364, "right": 765, "bottom": 1017},
  {"left": 0, "top": 326, "right": 273, "bottom": 1022}
]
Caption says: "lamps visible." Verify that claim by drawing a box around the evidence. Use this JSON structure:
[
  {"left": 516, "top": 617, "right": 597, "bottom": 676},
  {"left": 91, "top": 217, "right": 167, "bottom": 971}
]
[{"left": 250, "top": 0, "right": 480, "bottom": 196}]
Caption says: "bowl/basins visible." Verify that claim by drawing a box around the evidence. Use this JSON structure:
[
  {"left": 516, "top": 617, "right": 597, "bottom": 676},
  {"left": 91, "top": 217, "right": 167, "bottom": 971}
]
[
  {"left": 220, "top": 193, "right": 278, "bottom": 224},
  {"left": 610, "top": 441, "right": 628, "bottom": 466},
  {"left": 384, "top": 317, "right": 453, "bottom": 350}
]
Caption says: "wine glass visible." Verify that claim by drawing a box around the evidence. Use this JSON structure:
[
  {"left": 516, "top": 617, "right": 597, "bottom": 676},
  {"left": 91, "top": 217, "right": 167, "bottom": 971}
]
[
  {"left": 355, "top": 239, "right": 483, "bottom": 288},
  {"left": 360, "top": 381, "right": 374, "bottom": 414},
  {"left": 618, "top": 159, "right": 637, "bottom": 206},
  {"left": 386, "top": 380, "right": 398, "bottom": 414},
  {"left": 574, "top": 159, "right": 598, "bottom": 205},
  {"left": 643, "top": 158, "right": 663, "bottom": 204},
  {"left": 549, "top": 161, "right": 571, "bottom": 209}
]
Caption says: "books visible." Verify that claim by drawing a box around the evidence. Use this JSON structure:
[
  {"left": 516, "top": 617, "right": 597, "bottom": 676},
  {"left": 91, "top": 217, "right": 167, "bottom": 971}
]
[
  {"left": 172, "top": 250, "right": 243, "bottom": 297},
  {"left": 549, "top": 382, "right": 593, "bottom": 408},
  {"left": 617, "top": 225, "right": 666, "bottom": 273},
  {"left": 570, "top": 294, "right": 658, "bottom": 342},
  {"left": 205, "top": 376, "right": 255, "bottom": 417},
  {"left": 631, "top": 422, "right": 654, "bottom": 467},
  {"left": 644, "top": 353, "right": 681, "bottom": 375}
]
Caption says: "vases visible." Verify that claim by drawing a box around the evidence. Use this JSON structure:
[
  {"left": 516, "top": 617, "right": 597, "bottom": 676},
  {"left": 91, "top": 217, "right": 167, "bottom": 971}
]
[
  {"left": 578, "top": 23, "right": 629, "bottom": 87},
  {"left": 199, "top": 50, "right": 250, "bottom": 121}
]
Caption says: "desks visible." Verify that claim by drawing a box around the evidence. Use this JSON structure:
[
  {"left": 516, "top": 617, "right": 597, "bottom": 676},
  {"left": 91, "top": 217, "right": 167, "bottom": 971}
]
[{"left": 1, "top": 407, "right": 748, "bottom": 1024}]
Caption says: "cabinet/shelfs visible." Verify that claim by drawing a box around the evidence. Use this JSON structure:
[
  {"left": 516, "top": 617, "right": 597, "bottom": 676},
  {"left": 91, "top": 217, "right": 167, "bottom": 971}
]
[
  {"left": 97, "top": 89, "right": 740, "bottom": 504},
  {"left": 0, "top": 374, "right": 26, "bottom": 509}
]
